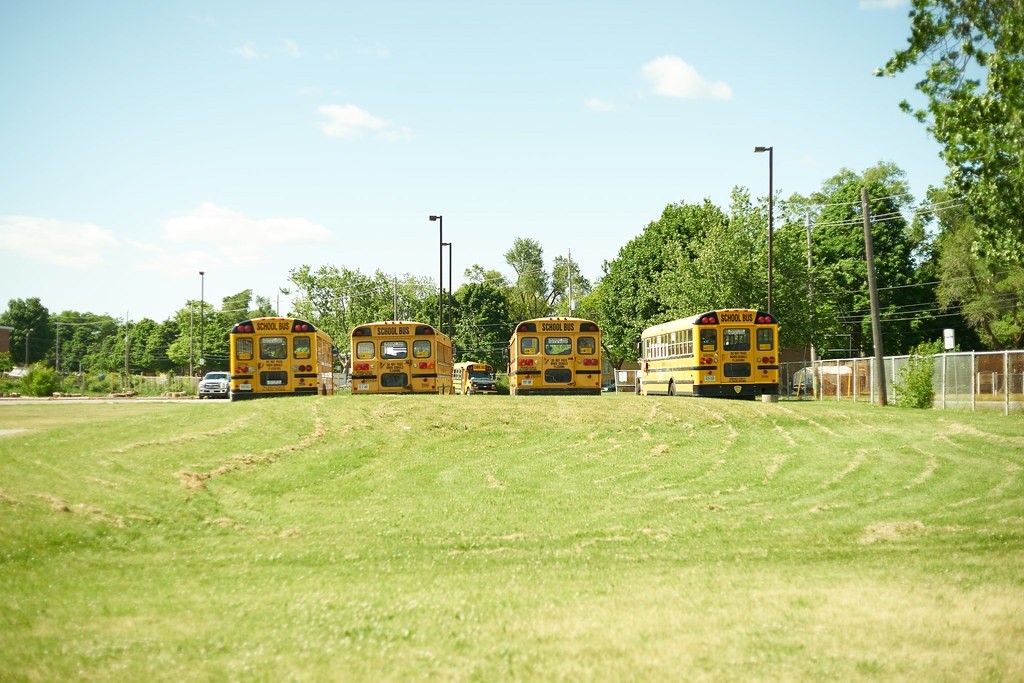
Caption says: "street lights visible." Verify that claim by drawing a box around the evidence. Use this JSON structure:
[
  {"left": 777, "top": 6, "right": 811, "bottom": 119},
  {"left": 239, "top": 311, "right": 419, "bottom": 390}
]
[
  {"left": 753, "top": 145, "right": 775, "bottom": 316},
  {"left": 442, "top": 242, "right": 451, "bottom": 341},
  {"left": 25, "top": 328, "right": 34, "bottom": 368},
  {"left": 199, "top": 271, "right": 205, "bottom": 381},
  {"left": 429, "top": 215, "right": 443, "bottom": 335}
]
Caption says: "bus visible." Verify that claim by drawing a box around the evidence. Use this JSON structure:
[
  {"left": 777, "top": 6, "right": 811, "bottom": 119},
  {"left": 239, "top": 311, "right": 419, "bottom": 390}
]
[
  {"left": 502, "top": 317, "right": 602, "bottom": 396},
  {"left": 461, "top": 362, "right": 498, "bottom": 395},
  {"left": 227, "top": 317, "right": 338, "bottom": 401},
  {"left": 639, "top": 308, "right": 781, "bottom": 398},
  {"left": 350, "top": 320, "right": 456, "bottom": 395}
]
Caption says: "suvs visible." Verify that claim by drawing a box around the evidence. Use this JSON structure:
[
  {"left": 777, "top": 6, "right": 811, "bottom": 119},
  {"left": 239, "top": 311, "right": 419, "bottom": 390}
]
[{"left": 198, "top": 371, "right": 231, "bottom": 399}]
